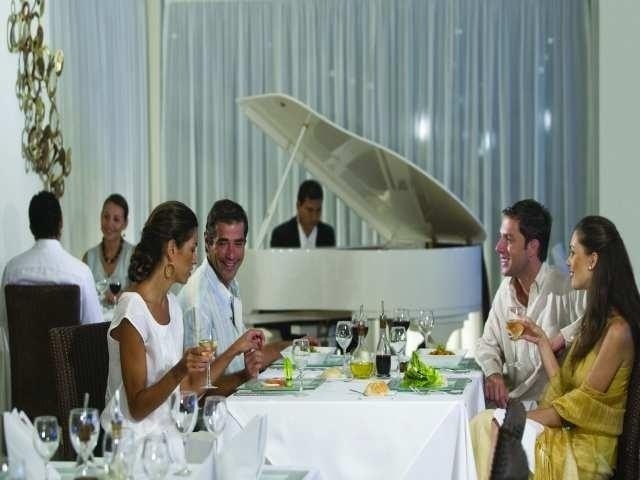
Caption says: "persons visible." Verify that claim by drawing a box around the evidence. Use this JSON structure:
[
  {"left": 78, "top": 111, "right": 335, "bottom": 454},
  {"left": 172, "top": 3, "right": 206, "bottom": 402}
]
[
  {"left": 177, "top": 199, "right": 321, "bottom": 408},
  {"left": 0, "top": 191, "right": 102, "bottom": 328},
  {"left": 82, "top": 195, "right": 139, "bottom": 309},
  {"left": 270, "top": 180, "right": 335, "bottom": 337},
  {"left": 470, "top": 216, "right": 640, "bottom": 480},
  {"left": 474, "top": 200, "right": 589, "bottom": 409},
  {"left": 104, "top": 201, "right": 265, "bottom": 443}
]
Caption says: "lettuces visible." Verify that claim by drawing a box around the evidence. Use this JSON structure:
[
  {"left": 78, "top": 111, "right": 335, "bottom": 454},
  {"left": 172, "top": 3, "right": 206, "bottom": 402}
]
[{"left": 403, "top": 352, "right": 448, "bottom": 392}]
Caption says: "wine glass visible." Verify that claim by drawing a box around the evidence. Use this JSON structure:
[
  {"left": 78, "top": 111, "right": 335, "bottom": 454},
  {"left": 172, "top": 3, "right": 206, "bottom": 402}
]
[
  {"left": 68, "top": 404, "right": 104, "bottom": 479},
  {"left": 503, "top": 305, "right": 527, "bottom": 369},
  {"left": 290, "top": 337, "right": 311, "bottom": 390},
  {"left": 110, "top": 280, "right": 121, "bottom": 308},
  {"left": 105, "top": 386, "right": 229, "bottom": 479},
  {"left": 32, "top": 416, "right": 62, "bottom": 480},
  {"left": 198, "top": 338, "right": 220, "bottom": 391},
  {"left": 93, "top": 269, "right": 112, "bottom": 306}
]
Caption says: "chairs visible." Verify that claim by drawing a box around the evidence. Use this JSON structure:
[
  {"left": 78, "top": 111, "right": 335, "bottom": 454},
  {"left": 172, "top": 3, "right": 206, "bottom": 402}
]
[
  {"left": 48, "top": 318, "right": 113, "bottom": 460},
  {"left": 613, "top": 333, "right": 639, "bottom": 479},
  {"left": 4, "top": 282, "right": 81, "bottom": 460}
]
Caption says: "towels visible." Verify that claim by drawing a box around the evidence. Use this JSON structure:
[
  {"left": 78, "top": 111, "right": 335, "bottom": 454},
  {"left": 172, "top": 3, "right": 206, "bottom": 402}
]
[
  {"left": 2, "top": 403, "right": 63, "bottom": 480},
  {"left": 194, "top": 411, "right": 269, "bottom": 480}
]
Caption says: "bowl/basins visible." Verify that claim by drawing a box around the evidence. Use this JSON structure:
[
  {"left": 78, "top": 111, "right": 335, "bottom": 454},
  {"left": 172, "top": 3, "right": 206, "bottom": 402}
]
[
  {"left": 281, "top": 344, "right": 329, "bottom": 367},
  {"left": 419, "top": 346, "right": 469, "bottom": 369}
]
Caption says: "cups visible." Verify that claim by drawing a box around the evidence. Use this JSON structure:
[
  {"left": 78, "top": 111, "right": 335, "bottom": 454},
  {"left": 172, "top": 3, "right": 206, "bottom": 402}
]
[
  {"left": 1, "top": 455, "right": 26, "bottom": 480},
  {"left": 335, "top": 299, "right": 435, "bottom": 383}
]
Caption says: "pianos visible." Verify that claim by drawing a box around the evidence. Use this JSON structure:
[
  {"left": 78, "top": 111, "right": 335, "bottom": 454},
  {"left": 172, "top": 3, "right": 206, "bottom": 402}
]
[{"left": 235, "top": 92, "right": 486, "bottom": 350}]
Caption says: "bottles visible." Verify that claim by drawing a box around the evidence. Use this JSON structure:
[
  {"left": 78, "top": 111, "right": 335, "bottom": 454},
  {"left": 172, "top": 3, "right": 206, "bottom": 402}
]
[
  {"left": 283, "top": 357, "right": 292, "bottom": 386},
  {"left": 285, "top": 376, "right": 292, "bottom": 387}
]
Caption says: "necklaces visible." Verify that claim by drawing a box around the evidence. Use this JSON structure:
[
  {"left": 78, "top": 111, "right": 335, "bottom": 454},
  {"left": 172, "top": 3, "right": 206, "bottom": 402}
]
[{"left": 102, "top": 236, "right": 123, "bottom": 264}]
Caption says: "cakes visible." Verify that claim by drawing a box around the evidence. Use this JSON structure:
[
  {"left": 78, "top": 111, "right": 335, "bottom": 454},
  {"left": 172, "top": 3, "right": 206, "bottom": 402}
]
[{"left": 365, "top": 381, "right": 389, "bottom": 395}]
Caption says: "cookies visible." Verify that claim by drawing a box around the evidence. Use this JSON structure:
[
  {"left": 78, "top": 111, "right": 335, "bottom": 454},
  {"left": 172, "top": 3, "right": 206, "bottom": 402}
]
[{"left": 262, "top": 377, "right": 283, "bottom": 390}]
